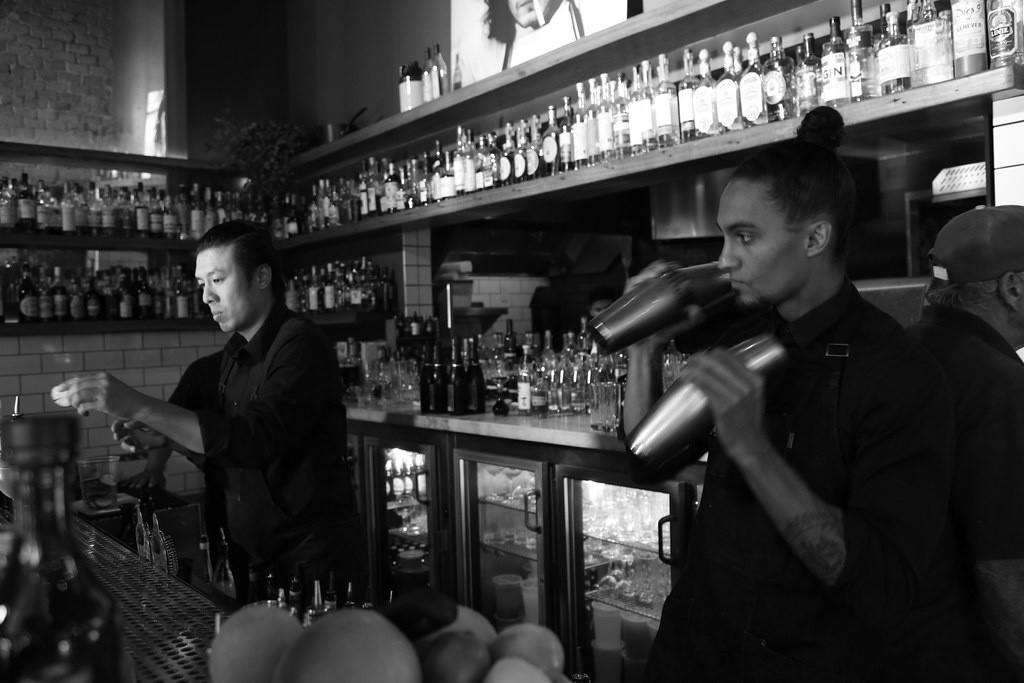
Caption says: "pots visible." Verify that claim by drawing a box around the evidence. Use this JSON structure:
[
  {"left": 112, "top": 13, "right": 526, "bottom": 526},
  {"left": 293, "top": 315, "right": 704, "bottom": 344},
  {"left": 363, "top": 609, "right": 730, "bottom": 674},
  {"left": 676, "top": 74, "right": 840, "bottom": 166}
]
[{"left": 312, "top": 107, "right": 369, "bottom": 146}]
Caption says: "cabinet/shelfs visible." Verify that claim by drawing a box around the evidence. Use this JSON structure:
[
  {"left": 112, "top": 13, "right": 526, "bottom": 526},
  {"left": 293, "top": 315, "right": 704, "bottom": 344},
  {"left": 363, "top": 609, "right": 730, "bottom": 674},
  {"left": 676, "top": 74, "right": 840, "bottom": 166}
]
[{"left": 0, "top": 0, "right": 1024, "bottom": 683}]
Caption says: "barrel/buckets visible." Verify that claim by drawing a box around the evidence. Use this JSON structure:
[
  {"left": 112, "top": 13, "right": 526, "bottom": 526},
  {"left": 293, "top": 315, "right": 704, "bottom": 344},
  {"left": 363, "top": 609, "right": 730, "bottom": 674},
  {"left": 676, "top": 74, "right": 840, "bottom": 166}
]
[{"left": 0, "top": 413, "right": 80, "bottom": 500}]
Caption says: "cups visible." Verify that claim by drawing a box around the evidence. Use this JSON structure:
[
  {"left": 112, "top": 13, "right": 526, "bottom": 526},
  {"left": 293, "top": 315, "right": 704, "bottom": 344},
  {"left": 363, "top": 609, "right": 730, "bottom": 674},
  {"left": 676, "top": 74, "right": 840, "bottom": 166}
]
[
  {"left": 585, "top": 260, "right": 734, "bottom": 351},
  {"left": 585, "top": 540, "right": 671, "bottom": 605},
  {"left": 493, "top": 561, "right": 540, "bottom": 634},
  {"left": 483, "top": 506, "right": 539, "bottom": 550},
  {"left": 592, "top": 380, "right": 620, "bottom": 432},
  {"left": 78, "top": 456, "right": 119, "bottom": 509},
  {"left": 398, "top": 61, "right": 424, "bottom": 114},
  {"left": 629, "top": 336, "right": 789, "bottom": 482},
  {"left": 591, "top": 601, "right": 660, "bottom": 683}
]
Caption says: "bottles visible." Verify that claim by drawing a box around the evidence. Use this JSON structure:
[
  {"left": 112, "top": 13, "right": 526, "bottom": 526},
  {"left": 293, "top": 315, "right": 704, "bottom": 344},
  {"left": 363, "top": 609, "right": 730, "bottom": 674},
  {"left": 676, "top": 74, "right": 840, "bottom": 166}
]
[
  {"left": 422, "top": 42, "right": 450, "bottom": 102},
  {"left": 0, "top": 447, "right": 134, "bottom": 683},
  {"left": 339, "top": 312, "right": 684, "bottom": 415},
  {"left": 0, "top": 258, "right": 213, "bottom": 323},
  {"left": 382, "top": 459, "right": 423, "bottom": 499},
  {"left": 286, "top": 257, "right": 399, "bottom": 315},
  {"left": 396, "top": 105, "right": 562, "bottom": 212},
  {"left": 560, "top": 0, "right": 1024, "bottom": 172},
  {"left": 267, "top": 157, "right": 400, "bottom": 242},
  {"left": 197, "top": 530, "right": 376, "bottom": 630},
  {"left": 0, "top": 174, "right": 266, "bottom": 243}
]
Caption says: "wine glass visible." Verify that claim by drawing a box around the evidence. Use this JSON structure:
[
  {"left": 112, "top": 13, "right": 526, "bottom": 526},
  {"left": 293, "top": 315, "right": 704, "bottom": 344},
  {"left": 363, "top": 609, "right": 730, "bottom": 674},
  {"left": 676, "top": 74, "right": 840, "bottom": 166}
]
[
  {"left": 394, "top": 490, "right": 416, "bottom": 533},
  {"left": 483, "top": 465, "right": 522, "bottom": 504},
  {"left": 368, "top": 362, "right": 392, "bottom": 406},
  {"left": 489, "top": 356, "right": 510, "bottom": 416}
]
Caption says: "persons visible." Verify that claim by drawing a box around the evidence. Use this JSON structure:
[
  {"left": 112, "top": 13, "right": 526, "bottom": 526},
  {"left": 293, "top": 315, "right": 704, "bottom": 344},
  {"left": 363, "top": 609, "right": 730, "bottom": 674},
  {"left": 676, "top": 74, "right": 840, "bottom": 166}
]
[
  {"left": 460, "top": 0, "right": 619, "bottom": 80},
  {"left": 621, "top": 106, "right": 1024, "bottom": 683},
  {"left": 57, "top": 219, "right": 356, "bottom": 605}
]
